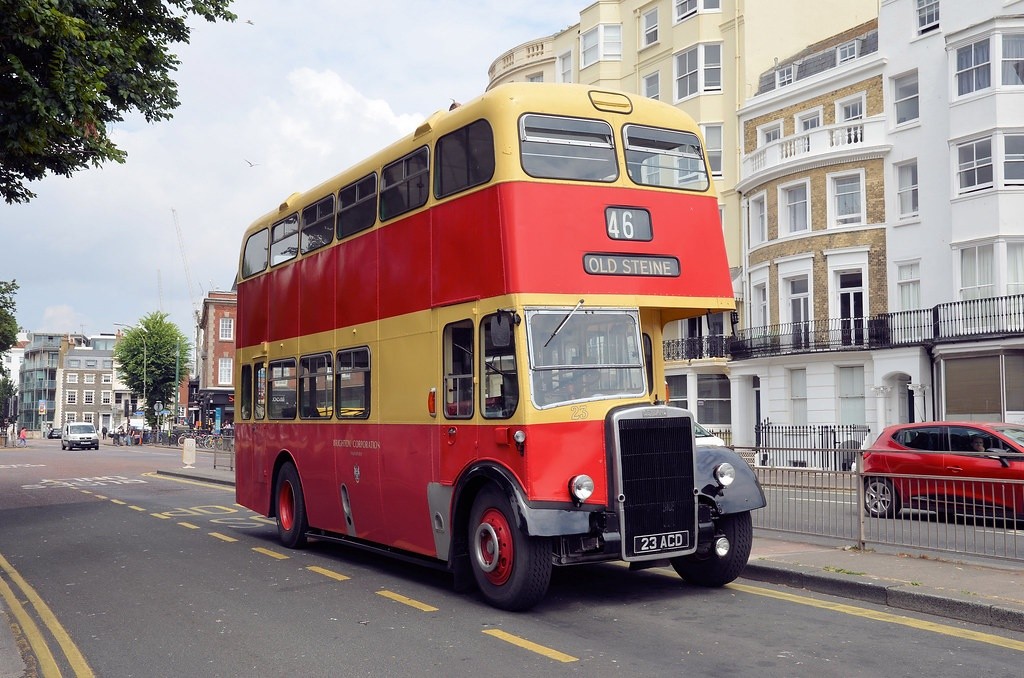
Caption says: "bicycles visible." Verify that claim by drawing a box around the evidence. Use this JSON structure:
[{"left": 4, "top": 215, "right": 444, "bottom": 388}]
[{"left": 177, "top": 433, "right": 232, "bottom": 452}]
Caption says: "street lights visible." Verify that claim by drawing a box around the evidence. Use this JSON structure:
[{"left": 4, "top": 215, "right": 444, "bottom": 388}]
[
  {"left": 173, "top": 336, "right": 180, "bottom": 445},
  {"left": 112, "top": 322, "right": 146, "bottom": 445}
]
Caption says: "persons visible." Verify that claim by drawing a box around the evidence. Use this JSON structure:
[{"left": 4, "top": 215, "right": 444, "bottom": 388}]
[
  {"left": 206, "top": 420, "right": 234, "bottom": 437},
  {"left": 102, "top": 425, "right": 142, "bottom": 446},
  {"left": 969, "top": 435, "right": 985, "bottom": 458},
  {"left": 15, "top": 426, "right": 28, "bottom": 448}
]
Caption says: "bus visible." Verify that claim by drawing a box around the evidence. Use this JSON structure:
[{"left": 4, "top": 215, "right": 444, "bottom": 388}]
[{"left": 233, "top": 81, "right": 768, "bottom": 611}]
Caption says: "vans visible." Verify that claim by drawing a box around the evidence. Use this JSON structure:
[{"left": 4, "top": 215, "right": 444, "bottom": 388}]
[{"left": 61, "top": 422, "right": 99, "bottom": 450}]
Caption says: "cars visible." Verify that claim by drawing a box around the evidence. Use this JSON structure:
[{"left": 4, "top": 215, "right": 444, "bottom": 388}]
[
  {"left": 47, "top": 429, "right": 62, "bottom": 438},
  {"left": 856, "top": 421, "right": 1024, "bottom": 523},
  {"left": 109, "top": 424, "right": 140, "bottom": 438}
]
